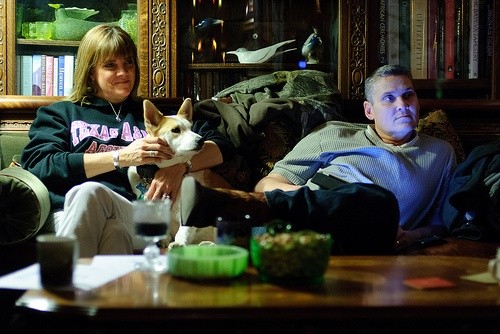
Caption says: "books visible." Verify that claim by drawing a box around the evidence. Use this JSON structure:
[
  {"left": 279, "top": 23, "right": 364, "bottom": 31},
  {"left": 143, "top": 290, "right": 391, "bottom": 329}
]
[
  {"left": 183, "top": 68, "right": 248, "bottom": 106},
  {"left": 367, "top": 1, "right": 494, "bottom": 102},
  {"left": 16, "top": 55, "right": 78, "bottom": 97}
]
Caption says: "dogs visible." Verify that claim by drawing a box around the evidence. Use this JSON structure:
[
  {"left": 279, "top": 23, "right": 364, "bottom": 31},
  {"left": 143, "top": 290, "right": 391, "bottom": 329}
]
[{"left": 128, "top": 97, "right": 265, "bottom": 251}]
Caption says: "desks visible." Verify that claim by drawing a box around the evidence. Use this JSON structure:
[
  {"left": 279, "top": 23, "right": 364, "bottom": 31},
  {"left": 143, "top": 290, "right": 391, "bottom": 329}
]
[{"left": 15, "top": 256, "right": 500, "bottom": 334}]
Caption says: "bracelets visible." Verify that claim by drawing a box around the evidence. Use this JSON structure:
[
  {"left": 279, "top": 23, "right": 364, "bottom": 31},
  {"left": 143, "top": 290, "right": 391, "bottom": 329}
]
[
  {"left": 185, "top": 160, "right": 192, "bottom": 174},
  {"left": 113, "top": 149, "right": 122, "bottom": 169}
]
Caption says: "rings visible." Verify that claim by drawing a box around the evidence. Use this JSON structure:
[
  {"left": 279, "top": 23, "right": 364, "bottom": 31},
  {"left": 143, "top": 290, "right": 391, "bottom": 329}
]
[{"left": 163, "top": 194, "right": 170, "bottom": 198}]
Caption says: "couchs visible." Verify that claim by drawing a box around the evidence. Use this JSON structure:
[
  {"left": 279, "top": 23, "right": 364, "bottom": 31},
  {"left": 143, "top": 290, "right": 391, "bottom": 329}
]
[{"left": 0, "top": 115, "right": 500, "bottom": 256}]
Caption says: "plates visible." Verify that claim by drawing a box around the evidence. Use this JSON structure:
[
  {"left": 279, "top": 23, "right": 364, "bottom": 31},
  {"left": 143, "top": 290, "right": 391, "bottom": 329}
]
[{"left": 167, "top": 245, "right": 248, "bottom": 281}]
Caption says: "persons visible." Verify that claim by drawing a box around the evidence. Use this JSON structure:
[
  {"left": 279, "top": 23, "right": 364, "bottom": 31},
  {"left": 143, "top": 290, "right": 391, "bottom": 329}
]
[
  {"left": 177, "top": 63, "right": 457, "bottom": 256},
  {"left": 21, "top": 25, "right": 245, "bottom": 257}
]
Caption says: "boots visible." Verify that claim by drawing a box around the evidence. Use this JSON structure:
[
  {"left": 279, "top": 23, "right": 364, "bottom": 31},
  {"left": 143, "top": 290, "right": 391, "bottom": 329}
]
[{"left": 180, "top": 177, "right": 268, "bottom": 228}]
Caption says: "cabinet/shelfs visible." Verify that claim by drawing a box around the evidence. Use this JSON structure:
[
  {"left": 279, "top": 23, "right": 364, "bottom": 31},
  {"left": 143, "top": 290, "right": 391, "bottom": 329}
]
[{"left": 0, "top": 0, "right": 500, "bottom": 118}]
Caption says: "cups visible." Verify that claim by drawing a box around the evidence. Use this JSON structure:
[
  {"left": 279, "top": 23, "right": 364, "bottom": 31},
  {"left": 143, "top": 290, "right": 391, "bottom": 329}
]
[
  {"left": 488, "top": 249, "right": 500, "bottom": 286},
  {"left": 36, "top": 234, "right": 77, "bottom": 292},
  {"left": 35, "top": 22, "right": 52, "bottom": 40}
]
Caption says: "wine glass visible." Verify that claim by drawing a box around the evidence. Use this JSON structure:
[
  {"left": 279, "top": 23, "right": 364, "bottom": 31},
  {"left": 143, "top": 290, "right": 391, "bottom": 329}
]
[{"left": 133, "top": 198, "right": 171, "bottom": 276}]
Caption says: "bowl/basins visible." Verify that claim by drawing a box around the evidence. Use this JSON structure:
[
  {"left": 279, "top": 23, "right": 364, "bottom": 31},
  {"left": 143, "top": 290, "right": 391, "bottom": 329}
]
[{"left": 250, "top": 231, "right": 333, "bottom": 289}]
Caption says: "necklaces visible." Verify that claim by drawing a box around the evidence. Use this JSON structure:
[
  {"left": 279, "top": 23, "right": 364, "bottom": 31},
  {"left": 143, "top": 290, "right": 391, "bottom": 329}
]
[{"left": 106, "top": 99, "right": 123, "bottom": 122}]
